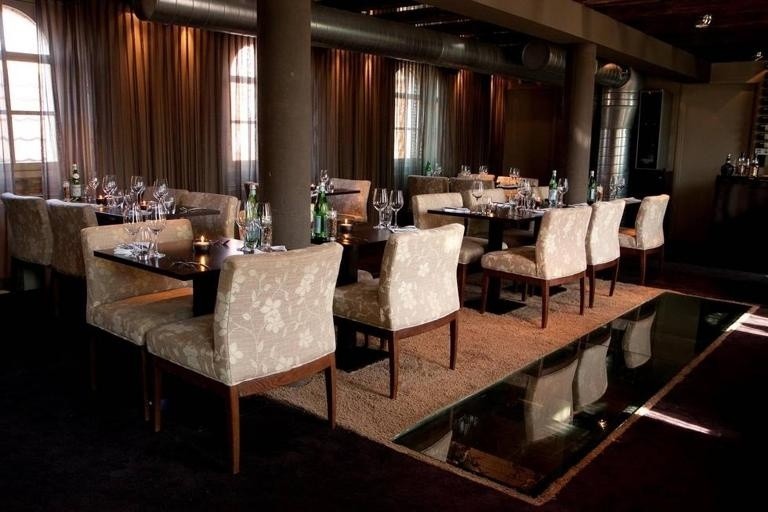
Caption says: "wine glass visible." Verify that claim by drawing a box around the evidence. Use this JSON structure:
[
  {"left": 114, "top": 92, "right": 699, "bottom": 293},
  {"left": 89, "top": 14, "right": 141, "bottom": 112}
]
[
  {"left": 235, "top": 201, "right": 272, "bottom": 250},
  {"left": 471, "top": 179, "right": 531, "bottom": 215},
  {"left": 557, "top": 178, "right": 568, "bottom": 205},
  {"left": 122, "top": 202, "right": 166, "bottom": 259},
  {"left": 371, "top": 189, "right": 404, "bottom": 230},
  {"left": 319, "top": 170, "right": 329, "bottom": 183},
  {"left": 87, "top": 175, "right": 169, "bottom": 206},
  {"left": 435, "top": 163, "right": 519, "bottom": 178},
  {"left": 609, "top": 176, "right": 626, "bottom": 196}
]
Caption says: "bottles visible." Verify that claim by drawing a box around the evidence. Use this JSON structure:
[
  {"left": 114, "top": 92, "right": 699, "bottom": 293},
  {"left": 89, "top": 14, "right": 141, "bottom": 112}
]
[
  {"left": 313, "top": 186, "right": 330, "bottom": 244},
  {"left": 720, "top": 153, "right": 734, "bottom": 176},
  {"left": 246, "top": 184, "right": 258, "bottom": 218},
  {"left": 424, "top": 161, "right": 431, "bottom": 176},
  {"left": 588, "top": 171, "right": 596, "bottom": 203},
  {"left": 70, "top": 163, "right": 81, "bottom": 200},
  {"left": 548, "top": 170, "right": 557, "bottom": 200}
]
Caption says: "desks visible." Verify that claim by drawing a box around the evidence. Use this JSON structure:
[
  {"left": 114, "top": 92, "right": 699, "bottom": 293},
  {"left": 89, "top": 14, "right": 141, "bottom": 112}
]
[
  {"left": 709, "top": 175, "right": 766, "bottom": 226},
  {"left": 427, "top": 191, "right": 642, "bottom": 315},
  {"left": 93, "top": 236, "right": 279, "bottom": 317},
  {"left": 308, "top": 187, "right": 360, "bottom": 196},
  {"left": 311, "top": 218, "right": 397, "bottom": 375}
]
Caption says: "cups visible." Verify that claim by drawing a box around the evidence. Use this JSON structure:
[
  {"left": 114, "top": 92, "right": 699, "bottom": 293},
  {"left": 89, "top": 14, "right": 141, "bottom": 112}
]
[
  {"left": 327, "top": 181, "right": 334, "bottom": 193},
  {"left": 62, "top": 181, "right": 69, "bottom": 202},
  {"left": 135, "top": 227, "right": 151, "bottom": 260},
  {"left": 734, "top": 158, "right": 759, "bottom": 176}
]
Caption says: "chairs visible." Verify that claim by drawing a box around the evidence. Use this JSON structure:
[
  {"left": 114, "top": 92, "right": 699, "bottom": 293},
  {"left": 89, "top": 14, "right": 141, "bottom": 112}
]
[
  {"left": 463, "top": 190, "right": 527, "bottom": 302},
  {"left": 326, "top": 177, "right": 373, "bottom": 224},
  {"left": 619, "top": 193, "right": 670, "bottom": 284},
  {"left": 146, "top": 242, "right": 344, "bottom": 473},
  {"left": 411, "top": 193, "right": 508, "bottom": 309},
  {"left": 2, "top": 184, "right": 241, "bottom": 318},
  {"left": 586, "top": 199, "right": 628, "bottom": 306},
  {"left": 334, "top": 221, "right": 466, "bottom": 400},
  {"left": 407, "top": 175, "right": 561, "bottom": 213},
  {"left": 480, "top": 204, "right": 592, "bottom": 327}
]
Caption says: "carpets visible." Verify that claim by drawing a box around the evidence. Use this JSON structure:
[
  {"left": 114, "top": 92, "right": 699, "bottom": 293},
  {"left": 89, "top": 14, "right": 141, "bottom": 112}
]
[{"left": 268, "top": 278, "right": 760, "bottom": 507}]
